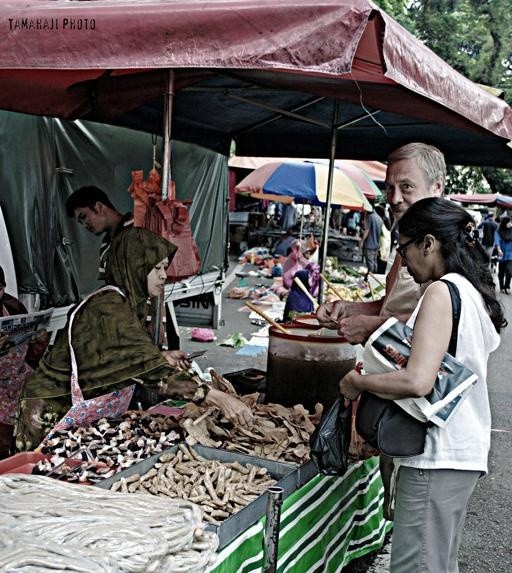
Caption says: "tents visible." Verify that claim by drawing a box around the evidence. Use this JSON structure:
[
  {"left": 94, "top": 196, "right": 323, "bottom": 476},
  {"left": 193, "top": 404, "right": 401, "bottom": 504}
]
[{"left": 0, "top": 0, "right": 512, "bottom": 306}]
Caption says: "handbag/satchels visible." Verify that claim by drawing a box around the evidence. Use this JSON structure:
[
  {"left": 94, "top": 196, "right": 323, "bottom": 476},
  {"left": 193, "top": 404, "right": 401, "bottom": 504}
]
[{"left": 354, "top": 279, "right": 461, "bottom": 457}]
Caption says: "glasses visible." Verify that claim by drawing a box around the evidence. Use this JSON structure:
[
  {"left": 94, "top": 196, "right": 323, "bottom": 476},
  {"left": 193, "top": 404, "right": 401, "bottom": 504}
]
[{"left": 395, "top": 235, "right": 424, "bottom": 256}]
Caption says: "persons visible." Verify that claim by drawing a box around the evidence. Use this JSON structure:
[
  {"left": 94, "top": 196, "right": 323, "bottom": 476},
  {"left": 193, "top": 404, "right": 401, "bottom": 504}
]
[
  {"left": 281, "top": 235, "right": 319, "bottom": 290},
  {"left": 330, "top": 203, "right": 363, "bottom": 237},
  {"left": 66, "top": 185, "right": 167, "bottom": 351},
  {"left": 267, "top": 198, "right": 322, "bottom": 235},
  {"left": 464, "top": 210, "right": 512, "bottom": 294},
  {"left": 338, "top": 197, "right": 508, "bottom": 572},
  {"left": 283, "top": 264, "right": 320, "bottom": 323},
  {"left": 11, "top": 226, "right": 259, "bottom": 457},
  {"left": 363, "top": 198, "right": 392, "bottom": 274},
  {"left": 315, "top": 142, "right": 446, "bottom": 347},
  {"left": 0, "top": 267, "right": 36, "bottom": 460}
]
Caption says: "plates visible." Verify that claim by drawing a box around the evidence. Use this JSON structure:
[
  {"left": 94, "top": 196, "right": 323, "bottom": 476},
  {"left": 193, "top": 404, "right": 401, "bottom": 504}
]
[
  {"left": 93, "top": 441, "right": 300, "bottom": 553},
  {"left": 193, "top": 443, "right": 320, "bottom": 489},
  {"left": 1, "top": 451, "right": 128, "bottom": 486}
]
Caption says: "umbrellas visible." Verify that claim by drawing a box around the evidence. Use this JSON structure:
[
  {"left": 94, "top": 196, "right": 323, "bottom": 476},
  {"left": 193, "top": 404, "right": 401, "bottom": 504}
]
[
  {"left": 234, "top": 160, "right": 373, "bottom": 244},
  {"left": 450, "top": 192, "right": 512, "bottom": 212},
  {"left": 304, "top": 159, "right": 382, "bottom": 238}
]
